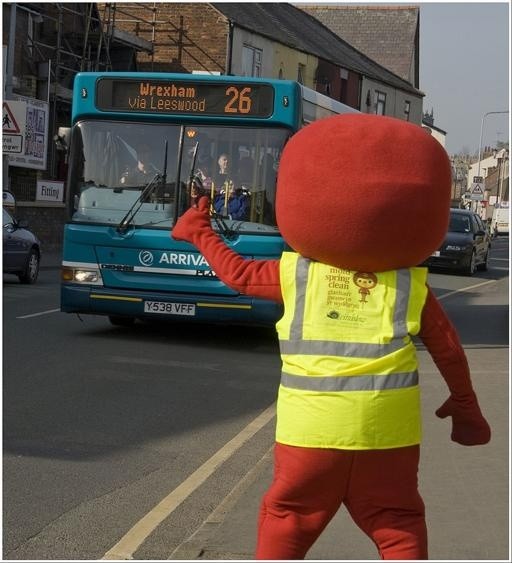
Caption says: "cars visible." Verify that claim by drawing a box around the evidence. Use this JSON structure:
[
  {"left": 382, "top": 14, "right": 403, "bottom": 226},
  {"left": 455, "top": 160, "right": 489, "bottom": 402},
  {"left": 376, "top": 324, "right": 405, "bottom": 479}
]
[
  {"left": 2, "top": 205, "right": 43, "bottom": 286},
  {"left": 418, "top": 206, "right": 495, "bottom": 278}
]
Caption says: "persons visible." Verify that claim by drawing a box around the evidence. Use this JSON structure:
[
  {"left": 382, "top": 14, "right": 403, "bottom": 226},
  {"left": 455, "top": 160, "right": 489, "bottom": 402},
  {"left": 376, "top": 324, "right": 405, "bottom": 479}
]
[{"left": 118, "top": 139, "right": 277, "bottom": 222}]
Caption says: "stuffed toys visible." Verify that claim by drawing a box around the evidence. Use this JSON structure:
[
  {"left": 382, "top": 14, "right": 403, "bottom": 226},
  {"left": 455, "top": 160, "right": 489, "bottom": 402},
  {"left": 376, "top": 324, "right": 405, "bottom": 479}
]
[{"left": 168, "top": 110, "right": 493, "bottom": 560}]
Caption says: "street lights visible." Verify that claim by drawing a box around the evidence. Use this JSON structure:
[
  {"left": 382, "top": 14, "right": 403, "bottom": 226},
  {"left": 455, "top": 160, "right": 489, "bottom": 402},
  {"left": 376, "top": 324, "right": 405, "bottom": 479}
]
[{"left": 491, "top": 145, "right": 507, "bottom": 204}]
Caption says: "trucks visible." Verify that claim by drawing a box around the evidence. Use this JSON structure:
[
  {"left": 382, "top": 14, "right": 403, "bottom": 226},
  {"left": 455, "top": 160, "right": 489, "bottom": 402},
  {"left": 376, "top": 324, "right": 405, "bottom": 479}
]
[{"left": 488, "top": 205, "right": 509, "bottom": 237}]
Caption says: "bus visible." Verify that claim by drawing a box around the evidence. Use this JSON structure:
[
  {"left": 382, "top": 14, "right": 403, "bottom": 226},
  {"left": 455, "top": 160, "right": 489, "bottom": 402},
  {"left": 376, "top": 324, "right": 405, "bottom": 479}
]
[{"left": 50, "top": 65, "right": 369, "bottom": 334}]
